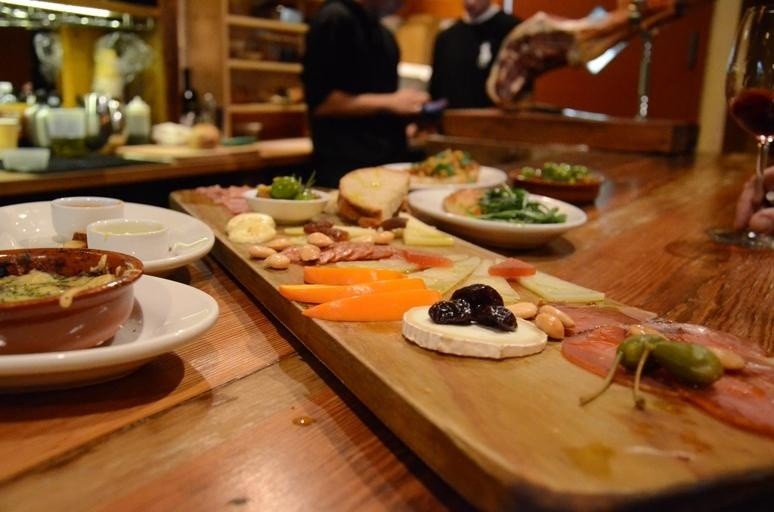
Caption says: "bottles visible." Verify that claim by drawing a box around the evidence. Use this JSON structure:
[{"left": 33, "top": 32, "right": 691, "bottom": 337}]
[
  {"left": 0, "top": 78, "right": 150, "bottom": 157},
  {"left": 179, "top": 68, "right": 201, "bottom": 123}
]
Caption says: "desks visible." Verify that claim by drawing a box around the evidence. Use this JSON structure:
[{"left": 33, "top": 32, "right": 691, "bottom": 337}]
[{"left": 0, "top": 138, "right": 316, "bottom": 200}]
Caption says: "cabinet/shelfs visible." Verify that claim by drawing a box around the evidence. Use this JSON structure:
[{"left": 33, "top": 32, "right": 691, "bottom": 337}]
[{"left": 175, "top": 0, "right": 305, "bottom": 137}]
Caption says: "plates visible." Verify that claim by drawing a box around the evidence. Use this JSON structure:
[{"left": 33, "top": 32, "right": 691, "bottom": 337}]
[
  {"left": 409, "top": 188, "right": 591, "bottom": 250},
  {"left": 0, "top": 271, "right": 219, "bottom": 395},
  {"left": 0, "top": 201, "right": 216, "bottom": 277},
  {"left": 380, "top": 161, "right": 506, "bottom": 188}
]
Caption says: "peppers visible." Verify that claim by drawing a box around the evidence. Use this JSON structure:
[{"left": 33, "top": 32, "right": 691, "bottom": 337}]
[{"left": 276, "top": 264, "right": 443, "bottom": 322}]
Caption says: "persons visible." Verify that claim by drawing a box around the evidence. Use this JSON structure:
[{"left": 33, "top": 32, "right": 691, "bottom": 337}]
[
  {"left": 303, "top": 1, "right": 435, "bottom": 189},
  {"left": 427, "top": 1, "right": 529, "bottom": 112},
  {"left": 730, "top": 163, "right": 774, "bottom": 239}
]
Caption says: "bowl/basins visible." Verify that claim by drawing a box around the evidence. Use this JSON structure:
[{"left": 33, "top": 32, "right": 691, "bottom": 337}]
[
  {"left": 245, "top": 187, "right": 331, "bottom": 225},
  {"left": 510, "top": 165, "right": 605, "bottom": 202},
  {"left": 86, "top": 217, "right": 169, "bottom": 260},
  {"left": 51, "top": 196, "right": 125, "bottom": 239},
  {"left": 0, "top": 245, "right": 142, "bottom": 354}
]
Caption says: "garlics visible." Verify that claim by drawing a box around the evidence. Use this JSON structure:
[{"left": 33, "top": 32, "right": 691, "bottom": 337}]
[
  {"left": 250, "top": 231, "right": 333, "bottom": 270},
  {"left": 505, "top": 302, "right": 574, "bottom": 341}
]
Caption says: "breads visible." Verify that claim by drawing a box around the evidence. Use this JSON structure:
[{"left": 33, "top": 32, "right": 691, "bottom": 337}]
[{"left": 336, "top": 167, "right": 410, "bottom": 224}]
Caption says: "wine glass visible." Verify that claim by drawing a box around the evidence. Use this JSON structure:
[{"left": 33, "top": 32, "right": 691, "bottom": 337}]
[{"left": 702, "top": 5, "right": 772, "bottom": 254}]
